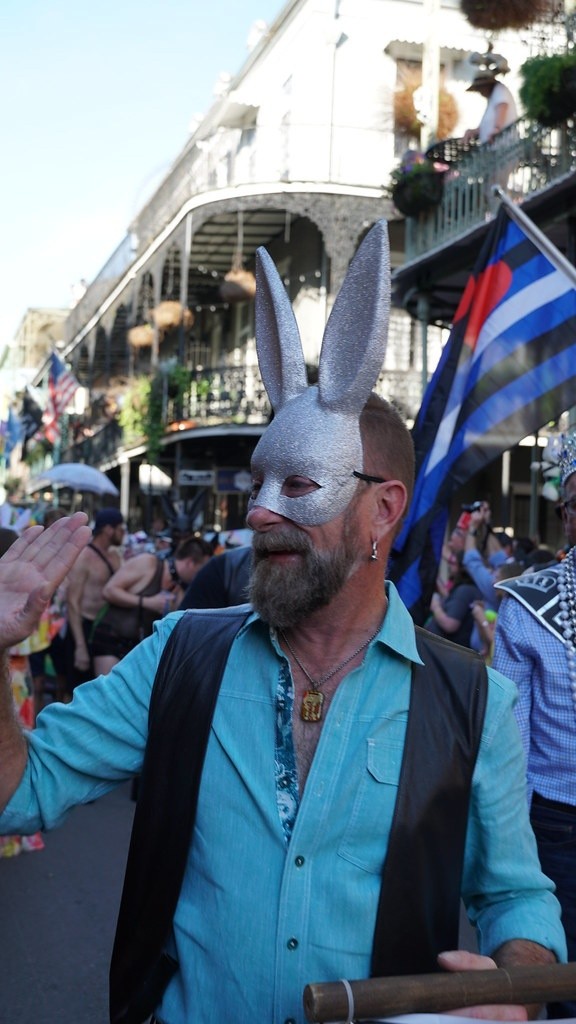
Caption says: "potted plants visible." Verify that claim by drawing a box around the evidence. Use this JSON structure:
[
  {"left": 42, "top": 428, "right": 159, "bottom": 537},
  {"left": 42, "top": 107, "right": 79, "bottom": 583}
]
[
  {"left": 518, "top": 55, "right": 576, "bottom": 127},
  {"left": 146, "top": 366, "right": 190, "bottom": 456}
]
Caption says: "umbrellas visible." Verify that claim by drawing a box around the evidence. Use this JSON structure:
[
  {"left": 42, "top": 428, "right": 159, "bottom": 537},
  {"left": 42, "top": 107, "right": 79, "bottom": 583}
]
[{"left": 34, "top": 457, "right": 119, "bottom": 513}]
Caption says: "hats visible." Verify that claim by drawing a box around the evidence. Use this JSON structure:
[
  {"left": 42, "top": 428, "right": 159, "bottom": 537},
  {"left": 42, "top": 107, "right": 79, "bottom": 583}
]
[
  {"left": 92, "top": 508, "right": 122, "bottom": 536},
  {"left": 559, "top": 432, "right": 576, "bottom": 487}
]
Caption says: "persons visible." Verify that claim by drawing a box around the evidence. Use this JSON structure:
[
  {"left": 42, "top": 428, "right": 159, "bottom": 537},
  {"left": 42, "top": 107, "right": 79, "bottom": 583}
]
[
  {"left": 425, "top": 501, "right": 568, "bottom": 652},
  {"left": 0, "top": 383, "right": 576, "bottom": 1021},
  {"left": 489, "top": 423, "right": 575, "bottom": 1020},
  {"left": 0, "top": 528, "right": 61, "bottom": 853},
  {"left": 26, "top": 509, "right": 73, "bottom": 722},
  {"left": 87, "top": 540, "right": 214, "bottom": 677},
  {"left": 460, "top": 66, "right": 517, "bottom": 203},
  {"left": 66, "top": 510, "right": 126, "bottom": 696}
]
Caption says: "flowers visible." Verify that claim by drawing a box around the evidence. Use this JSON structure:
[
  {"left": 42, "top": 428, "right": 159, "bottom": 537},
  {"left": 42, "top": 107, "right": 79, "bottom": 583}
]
[{"left": 388, "top": 149, "right": 448, "bottom": 181}]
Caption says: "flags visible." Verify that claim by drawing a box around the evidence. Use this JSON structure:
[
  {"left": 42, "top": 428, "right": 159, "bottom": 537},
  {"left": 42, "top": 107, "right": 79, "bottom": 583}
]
[
  {"left": 19, "top": 388, "right": 45, "bottom": 460},
  {"left": 0, "top": 395, "right": 20, "bottom": 472},
  {"left": 44, "top": 353, "right": 81, "bottom": 444},
  {"left": 373, "top": 205, "right": 576, "bottom": 625}
]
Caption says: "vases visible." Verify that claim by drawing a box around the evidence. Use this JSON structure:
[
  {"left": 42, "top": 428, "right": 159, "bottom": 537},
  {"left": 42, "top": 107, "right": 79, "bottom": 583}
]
[{"left": 392, "top": 176, "right": 444, "bottom": 216}]
